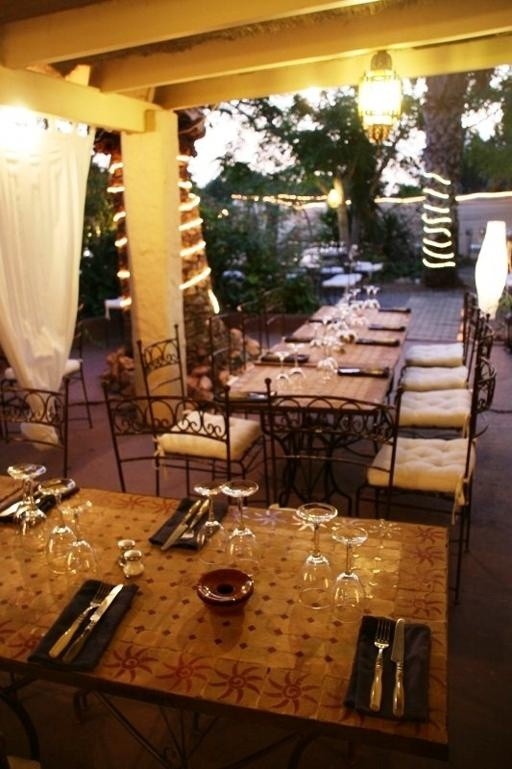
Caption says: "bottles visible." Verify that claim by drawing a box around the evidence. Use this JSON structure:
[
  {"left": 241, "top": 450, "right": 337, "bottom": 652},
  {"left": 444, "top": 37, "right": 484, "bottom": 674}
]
[{"left": 117, "top": 539, "right": 145, "bottom": 578}]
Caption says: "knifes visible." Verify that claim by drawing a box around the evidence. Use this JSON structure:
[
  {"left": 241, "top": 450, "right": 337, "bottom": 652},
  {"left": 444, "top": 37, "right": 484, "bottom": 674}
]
[
  {"left": 339, "top": 368, "right": 384, "bottom": 375},
  {"left": 62, "top": 583, "right": 124, "bottom": 665},
  {"left": 161, "top": 499, "right": 202, "bottom": 551},
  {"left": 391, "top": 618, "right": 406, "bottom": 716},
  {"left": 0, "top": 489, "right": 44, "bottom": 517}
]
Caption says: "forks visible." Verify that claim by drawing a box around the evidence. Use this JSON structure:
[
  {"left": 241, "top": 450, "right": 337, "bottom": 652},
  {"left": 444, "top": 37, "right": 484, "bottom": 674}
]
[
  {"left": 370, "top": 617, "right": 391, "bottom": 711},
  {"left": 176, "top": 498, "right": 210, "bottom": 545},
  {"left": 48, "top": 578, "right": 112, "bottom": 658}
]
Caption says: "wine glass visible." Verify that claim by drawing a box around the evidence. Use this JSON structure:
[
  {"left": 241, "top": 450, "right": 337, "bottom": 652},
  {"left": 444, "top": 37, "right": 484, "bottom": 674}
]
[
  {"left": 7, "top": 463, "right": 98, "bottom": 577},
  {"left": 295, "top": 502, "right": 369, "bottom": 625},
  {"left": 273, "top": 283, "right": 380, "bottom": 394},
  {"left": 193, "top": 479, "right": 261, "bottom": 577}
]
